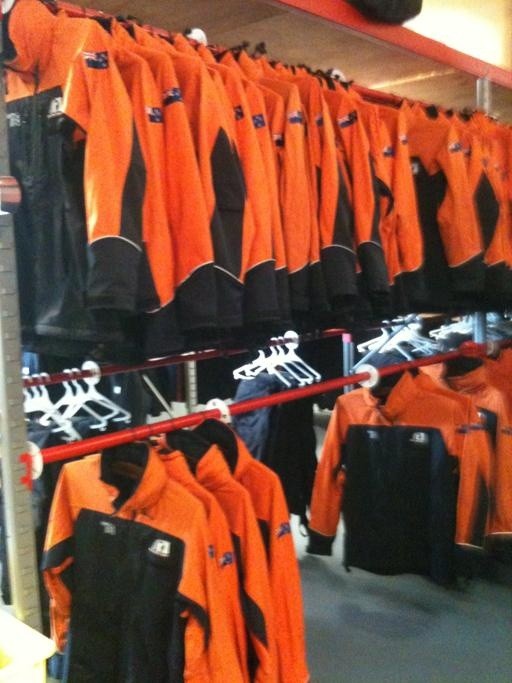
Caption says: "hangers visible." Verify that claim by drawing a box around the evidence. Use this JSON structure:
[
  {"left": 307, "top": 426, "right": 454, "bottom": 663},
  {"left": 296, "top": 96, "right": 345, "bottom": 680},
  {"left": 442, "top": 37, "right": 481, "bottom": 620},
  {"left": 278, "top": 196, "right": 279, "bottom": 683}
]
[{"left": 356, "top": 301, "right": 512, "bottom": 364}]
